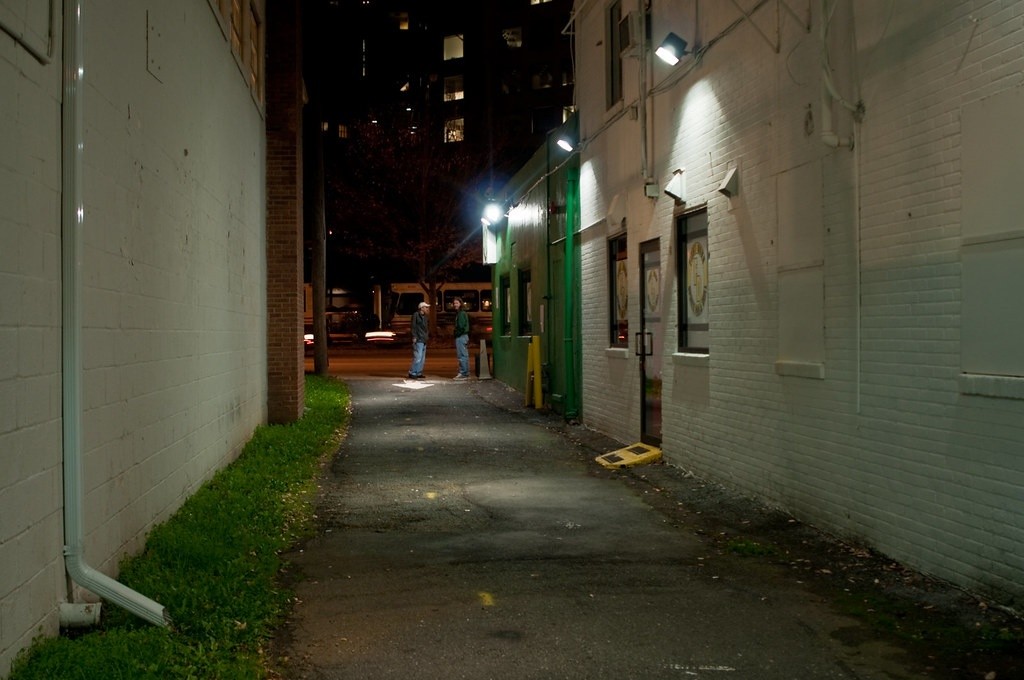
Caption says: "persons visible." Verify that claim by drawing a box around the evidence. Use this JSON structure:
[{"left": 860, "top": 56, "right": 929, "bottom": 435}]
[
  {"left": 408, "top": 302, "right": 430, "bottom": 380},
  {"left": 452, "top": 297, "right": 470, "bottom": 381}
]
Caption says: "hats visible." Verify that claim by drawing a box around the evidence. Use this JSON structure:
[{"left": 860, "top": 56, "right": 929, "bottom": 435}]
[{"left": 418, "top": 302, "right": 430, "bottom": 309}]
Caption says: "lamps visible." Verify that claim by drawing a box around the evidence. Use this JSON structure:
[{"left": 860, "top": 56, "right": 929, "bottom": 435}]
[
  {"left": 557, "top": 127, "right": 586, "bottom": 153},
  {"left": 655, "top": 32, "right": 698, "bottom": 67}
]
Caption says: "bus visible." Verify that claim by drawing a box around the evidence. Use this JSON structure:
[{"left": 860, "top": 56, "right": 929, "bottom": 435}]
[
  {"left": 302, "top": 280, "right": 360, "bottom": 346},
  {"left": 371, "top": 280, "right": 493, "bottom": 346}
]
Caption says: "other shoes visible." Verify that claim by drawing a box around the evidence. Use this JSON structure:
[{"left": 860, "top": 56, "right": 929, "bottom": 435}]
[
  {"left": 408, "top": 373, "right": 416, "bottom": 379},
  {"left": 453, "top": 373, "right": 468, "bottom": 380},
  {"left": 467, "top": 374, "right": 471, "bottom": 379},
  {"left": 417, "top": 375, "right": 425, "bottom": 378}
]
